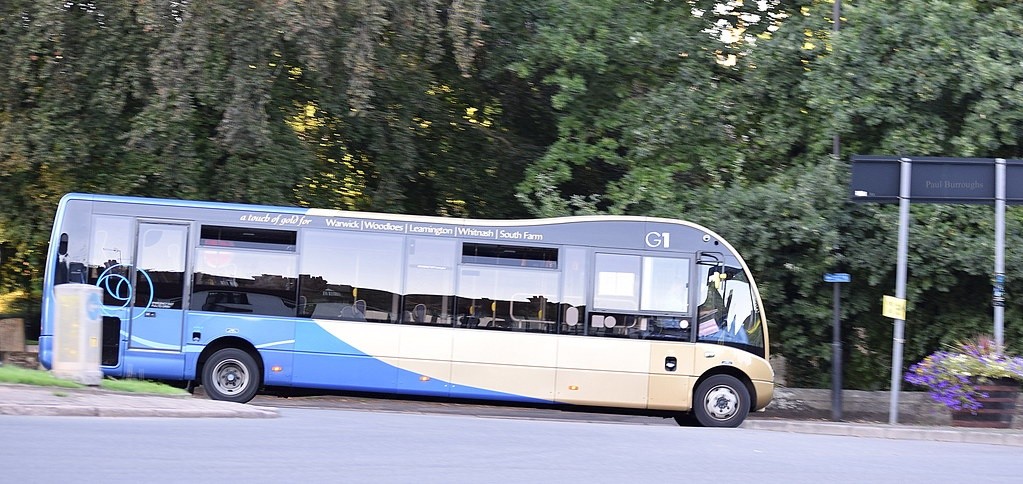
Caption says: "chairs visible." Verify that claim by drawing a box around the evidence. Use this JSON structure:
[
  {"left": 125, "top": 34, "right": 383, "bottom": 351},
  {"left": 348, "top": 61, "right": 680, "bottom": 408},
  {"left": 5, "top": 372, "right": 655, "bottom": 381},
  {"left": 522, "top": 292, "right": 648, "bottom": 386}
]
[
  {"left": 467, "top": 305, "right": 482, "bottom": 327},
  {"left": 339, "top": 299, "right": 366, "bottom": 318},
  {"left": 403, "top": 303, "right": 426, "bottom": 323},
  {"left": 298, "top": 296, "right": 308, "bottom": 316}
]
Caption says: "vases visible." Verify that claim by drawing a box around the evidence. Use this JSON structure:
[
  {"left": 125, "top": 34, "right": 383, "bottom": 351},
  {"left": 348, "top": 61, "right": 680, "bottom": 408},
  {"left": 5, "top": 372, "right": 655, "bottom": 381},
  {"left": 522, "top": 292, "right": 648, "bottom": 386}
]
[{"left": 953, "top": 376, "right": 1019, "bottom": 428}]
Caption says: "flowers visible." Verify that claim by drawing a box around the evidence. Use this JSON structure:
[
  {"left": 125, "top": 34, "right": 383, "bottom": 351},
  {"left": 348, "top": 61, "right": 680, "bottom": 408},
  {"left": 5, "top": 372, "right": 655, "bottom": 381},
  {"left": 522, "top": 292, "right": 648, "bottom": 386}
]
[{"left": 905, "top": 335, "right": 1023, "bottom": 415}]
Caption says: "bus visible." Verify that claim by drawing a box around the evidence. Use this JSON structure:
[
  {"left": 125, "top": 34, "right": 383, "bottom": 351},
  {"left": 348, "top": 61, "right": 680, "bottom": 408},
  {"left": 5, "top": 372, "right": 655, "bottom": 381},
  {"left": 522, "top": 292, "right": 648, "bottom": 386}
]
[{"left": 37, "top": 192, "right": 775, "bottom": 428}]
